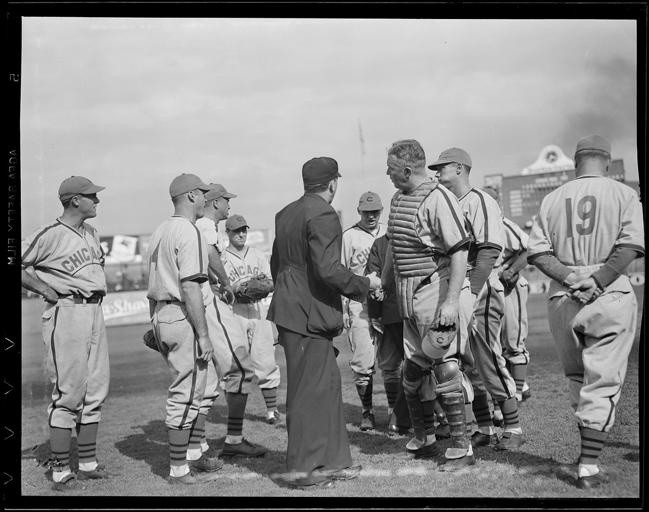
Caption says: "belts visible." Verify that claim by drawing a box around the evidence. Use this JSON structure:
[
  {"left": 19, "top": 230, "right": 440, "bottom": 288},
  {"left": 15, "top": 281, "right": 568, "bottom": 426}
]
[{"left": 73, "top": 293, "right": 104, "bottom": 302}]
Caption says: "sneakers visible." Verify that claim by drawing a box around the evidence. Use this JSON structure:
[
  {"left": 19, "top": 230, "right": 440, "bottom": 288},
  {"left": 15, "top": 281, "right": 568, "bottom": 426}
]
[
  {"left": 53, "top": 474, "right": 86, "bottom": 491},
  {"left": 438, "top": 455, "right": 476, "bottom": 472},
  {"left": 360, "top": 411, "right": 375, "bottom": 431},
  {"left": 267, "top": 416, "right": 284, "bottom": 427},
  {"left": 388, "top": 425, "right": 450, "bottom": 460},
  {"left": 298, "top": 463, "right": 363, "bottom": 490},
  {"left": 578, "top": 469, "right": 611, "bottom": 489},
  {"left": 77, "top": 465, "right": 112, "bottom": 479},
  {"left": 469, "top": 431, "right": 525, "bottom": 450},
  {"left": 222, "top": 438, "right": 266, "bottom": 456},
  {"left": 491, "top": 387, "right": 530, "bottom": 426},
  {"left": 168, "top": 447, "right": 223, "bottom": 483}
]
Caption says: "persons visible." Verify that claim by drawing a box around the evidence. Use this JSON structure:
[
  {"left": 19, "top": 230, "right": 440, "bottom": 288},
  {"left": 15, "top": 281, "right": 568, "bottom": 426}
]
[
  {"left": 267, "top": 157, "right": 381, "bottom": 489},
  {"left": 341, "top": 138, "right": 531, "bottom": 473},
  {"left": 147, "top": 174, "right": 224, "bottom": 485},
  {"left": 194, "top": 184, "right": 267, "bottom": 458},
  {"left": 21, "top": 176, "right": 110, "bottom": 491},
  {"left": 220, "top": 215, "right": 286, "bottom": 425},
  {"left": 527, "top": 136, "right": 645, "bottom": 490}
]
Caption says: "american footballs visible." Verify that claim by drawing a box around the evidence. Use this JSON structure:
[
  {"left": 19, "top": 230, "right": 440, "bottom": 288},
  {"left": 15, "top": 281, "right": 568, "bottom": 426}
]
[{"left": 244, "top": 278, "right": 270, "bottom": 299}]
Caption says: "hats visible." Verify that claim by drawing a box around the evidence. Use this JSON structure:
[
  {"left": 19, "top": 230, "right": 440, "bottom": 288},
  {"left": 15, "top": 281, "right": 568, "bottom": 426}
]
[
  {"left": 203, "top": 182, "right": 237, "bottom": 202},
  {"left": 169, "top": 173, "right": 210, "bottom": 200},
  {"left": 226, "top": 214, "right": 251, "bottom": 231},
  {"left": 427, "top": 147, "right": 472, "bottom": 170},
  {"left": 577, "top": 135, "right": 611, "bottom": 157},
  {"left": 59, "top": 176, "right": 106, "bottom": 202},
  {"left": 303, "top": 157, "right": 342, "bottom": 184},
  {"left": 421, "top": 318, "right": 458, "bottom": 359},
  {"left": 358, "top": 192, "right": 383, "bottom": 213}
]
